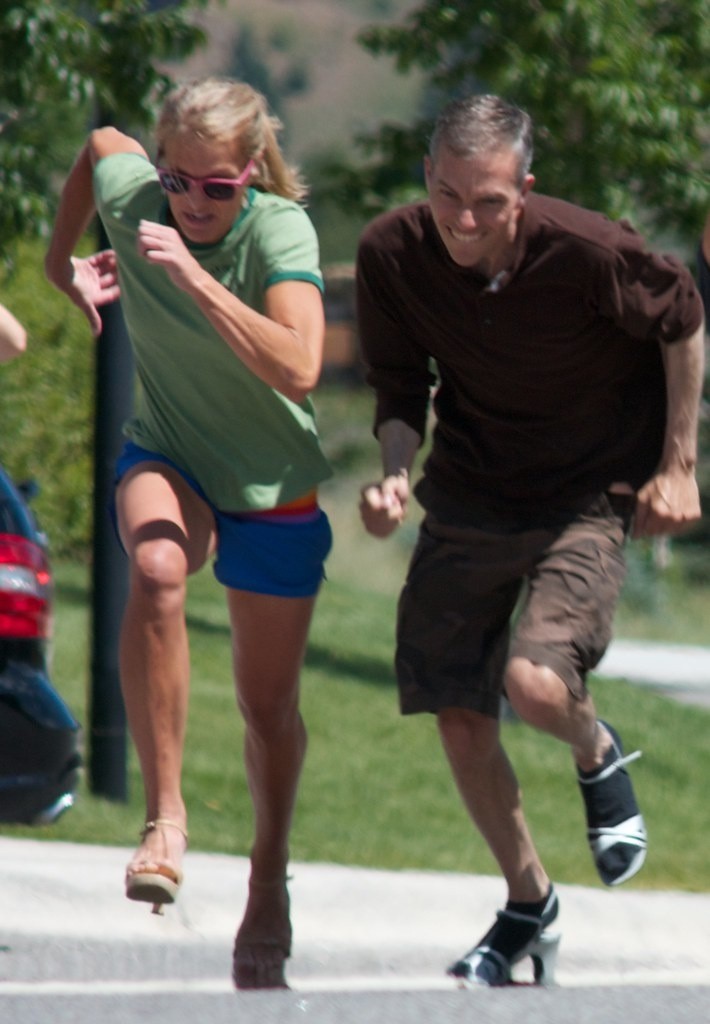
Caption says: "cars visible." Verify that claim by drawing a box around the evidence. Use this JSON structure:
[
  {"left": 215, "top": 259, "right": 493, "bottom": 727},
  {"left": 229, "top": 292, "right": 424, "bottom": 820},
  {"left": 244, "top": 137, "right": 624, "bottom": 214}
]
[{"left": 0, "top": 469, "right": 79, "bottom": 827}]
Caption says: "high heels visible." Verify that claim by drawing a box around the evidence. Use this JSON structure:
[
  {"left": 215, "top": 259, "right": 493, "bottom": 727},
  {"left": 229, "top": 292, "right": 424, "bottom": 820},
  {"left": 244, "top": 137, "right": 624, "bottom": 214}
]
[
  {"left": 126, "top": 819, "right": 189, "bottom": 914},
  {"left": 452, "top": 884, "right": 561, "bottom": 986},
  {"left": 576, "top": 719, "right": 648, "bottom": 885},
  {"left": 233, "top": 876, "right": 291, "bottom": 985}
]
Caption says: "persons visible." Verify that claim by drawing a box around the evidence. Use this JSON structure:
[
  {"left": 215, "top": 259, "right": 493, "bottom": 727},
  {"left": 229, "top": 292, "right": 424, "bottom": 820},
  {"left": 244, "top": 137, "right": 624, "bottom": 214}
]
[
  {"left": 46, "top": 79, "right": 331, "bottom": 991},
  {"left": 356, "top": 91, "right": 706, "bottom": 989}
]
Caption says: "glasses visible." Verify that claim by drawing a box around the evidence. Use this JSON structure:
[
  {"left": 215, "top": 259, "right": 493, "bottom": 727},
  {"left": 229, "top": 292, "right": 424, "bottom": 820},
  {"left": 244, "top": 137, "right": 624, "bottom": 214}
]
[{"left": 156, "top": 157, "right": 253, "bottom": 202}]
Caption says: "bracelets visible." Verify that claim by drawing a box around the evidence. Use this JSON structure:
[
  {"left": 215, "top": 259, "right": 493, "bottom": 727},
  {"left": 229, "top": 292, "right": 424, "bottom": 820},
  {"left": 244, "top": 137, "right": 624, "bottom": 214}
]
[{"left": 63, "top": 257, "right": 77, "bottom": 288}]
